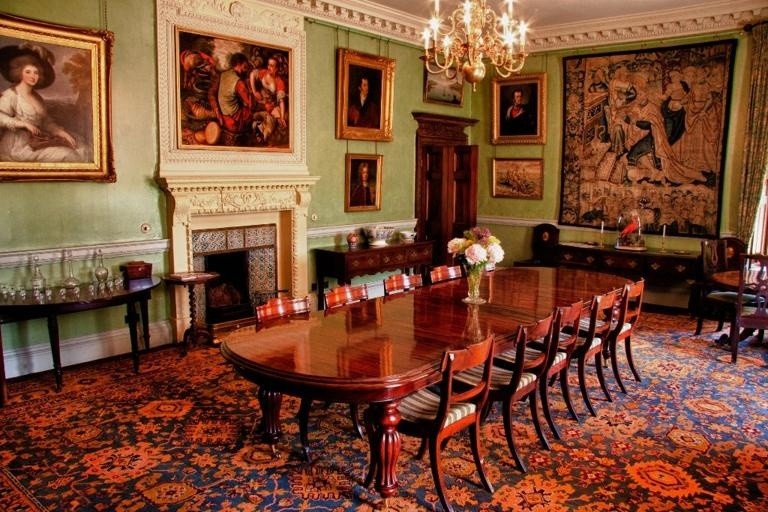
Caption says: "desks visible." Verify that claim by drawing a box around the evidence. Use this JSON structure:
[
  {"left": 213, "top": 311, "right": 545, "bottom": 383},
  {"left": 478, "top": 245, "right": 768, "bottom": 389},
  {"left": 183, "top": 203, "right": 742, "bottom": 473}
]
[
  {"left": 0, "top": 276, "right": 162, "bottom": 406},
  {"left": 311, "top": 238, "right": 434, "bottom": 311},
  {"left": 163, "top": 272, "right": 223, "bottom": 359},
  {"left": 546, "top": 240, "right": 702, "bottom": 321},
  {"left": 219, "top": 264, "right": 636, "bottom": 500}
]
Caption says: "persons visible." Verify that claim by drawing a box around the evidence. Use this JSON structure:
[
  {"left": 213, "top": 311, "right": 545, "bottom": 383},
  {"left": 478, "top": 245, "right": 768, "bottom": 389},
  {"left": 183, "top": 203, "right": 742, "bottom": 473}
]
[
  {"left": 0, "top": 55, "right": 82, "bottom": 164},
  {"left": 505, "top": 89, "right": 528, "bottom": 126},
  {"left": 208, "top": 51, "right": 287, "bottom": 145},
  {"left": 350, "top": 161, "right": 372, "bottom": 206},
  {"left": 348, "top": 76, "right": 377, "bottom": 128}
]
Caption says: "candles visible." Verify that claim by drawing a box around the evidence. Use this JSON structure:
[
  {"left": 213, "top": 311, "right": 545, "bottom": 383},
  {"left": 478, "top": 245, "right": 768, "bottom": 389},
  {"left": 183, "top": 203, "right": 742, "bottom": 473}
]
[
  {"left": 600, "top": 220, "right": 604, "bottom": 233},
  {"left": 662, "top": 224, "right": 667, "bottom": 238}
]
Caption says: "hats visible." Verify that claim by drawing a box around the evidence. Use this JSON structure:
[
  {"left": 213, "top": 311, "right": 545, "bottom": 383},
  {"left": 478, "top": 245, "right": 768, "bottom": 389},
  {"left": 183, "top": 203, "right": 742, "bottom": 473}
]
[{"left": 1, "top": 41, "right": 57, "bottom": 91}]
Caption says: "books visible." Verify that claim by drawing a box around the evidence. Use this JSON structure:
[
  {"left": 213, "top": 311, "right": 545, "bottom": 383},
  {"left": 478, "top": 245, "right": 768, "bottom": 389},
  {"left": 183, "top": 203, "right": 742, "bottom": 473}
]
[{"left": 170, "top": 272, "right": 215, "bottom": 282}]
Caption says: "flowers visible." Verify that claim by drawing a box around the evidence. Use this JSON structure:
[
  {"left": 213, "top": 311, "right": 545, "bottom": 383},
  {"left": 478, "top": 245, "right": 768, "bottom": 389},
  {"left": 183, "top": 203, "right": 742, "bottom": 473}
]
[{"left": 446, "top": 225, "right": 504, "bottom": 271}]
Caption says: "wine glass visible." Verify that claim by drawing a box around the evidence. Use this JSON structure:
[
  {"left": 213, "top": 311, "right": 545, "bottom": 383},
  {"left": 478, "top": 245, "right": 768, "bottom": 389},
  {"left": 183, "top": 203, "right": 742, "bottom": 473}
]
[{"left": 361, "top": 224, "right": 396, "bottom": 246}]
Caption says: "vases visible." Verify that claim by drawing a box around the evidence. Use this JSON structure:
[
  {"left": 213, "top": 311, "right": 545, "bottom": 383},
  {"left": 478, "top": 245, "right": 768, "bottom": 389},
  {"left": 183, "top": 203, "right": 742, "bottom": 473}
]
[
  {"left": 461, "top": 268, "right": 488, "bottom": 305},
  {"left": 460, "top": 304, "right": 486, "bottom": 343}
]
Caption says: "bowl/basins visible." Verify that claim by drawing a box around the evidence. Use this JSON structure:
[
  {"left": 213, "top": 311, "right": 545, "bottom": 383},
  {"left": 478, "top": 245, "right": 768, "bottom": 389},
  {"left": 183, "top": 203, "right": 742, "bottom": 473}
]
[{"left": 399, "top": 230, "right": 418, "bottom": 238}]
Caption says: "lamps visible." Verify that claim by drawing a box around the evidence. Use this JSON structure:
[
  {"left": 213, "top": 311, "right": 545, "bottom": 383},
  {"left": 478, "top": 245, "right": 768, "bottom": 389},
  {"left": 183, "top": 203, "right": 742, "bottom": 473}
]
[{"left": 419, "top": 0, "right": 529, "bottom": 93}]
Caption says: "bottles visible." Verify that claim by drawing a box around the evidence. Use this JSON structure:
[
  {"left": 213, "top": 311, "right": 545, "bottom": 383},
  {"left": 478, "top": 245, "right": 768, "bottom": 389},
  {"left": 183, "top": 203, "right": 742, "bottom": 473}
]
[{"left": 0, "top": 247, "right": 124, "bottom": 304}]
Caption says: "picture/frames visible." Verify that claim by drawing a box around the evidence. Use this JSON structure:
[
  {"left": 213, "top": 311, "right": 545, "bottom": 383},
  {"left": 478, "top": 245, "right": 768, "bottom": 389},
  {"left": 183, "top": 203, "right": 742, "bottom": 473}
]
[
  {"left": 422, "top": 61, "right": 465, "bottom": 108},
  {"left": 173, "top": 24, "right": 294, "bottom": 154},
  {"left": 345, "top": 153, "right": 384, "bottom": 212},
  {"left": 0, "top": 10, "right": 118, "bottom": 184},
  {"left": 491, "top": 157, "right": 544, "bottom": 200},
  {"left": 490, "top": 72, "right": 547, "bottom": 145},
  {"left": 335, "top": 47, "right": 397, "bottom": 142}
]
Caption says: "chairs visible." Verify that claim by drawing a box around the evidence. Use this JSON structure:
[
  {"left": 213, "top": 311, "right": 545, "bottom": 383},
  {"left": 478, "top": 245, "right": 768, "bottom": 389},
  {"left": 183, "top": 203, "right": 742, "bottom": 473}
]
[
  {"left": 688, "top": 236, "right": 768, "bottom": 363},
  {"left": 512, "top": 223, "right": 559, "bottom": 268}
]
